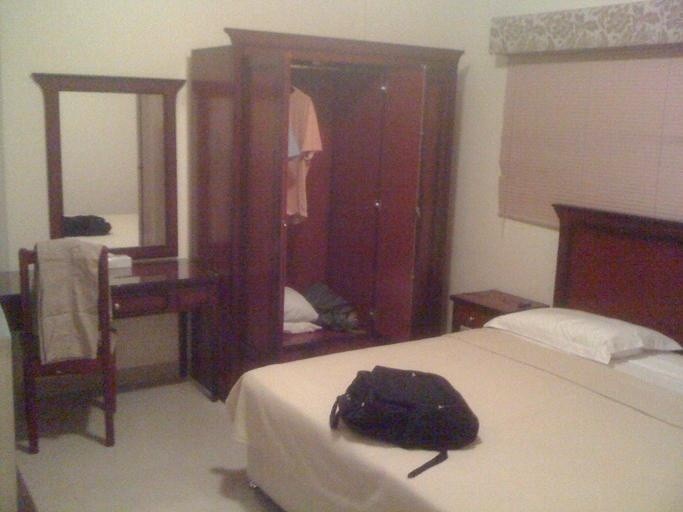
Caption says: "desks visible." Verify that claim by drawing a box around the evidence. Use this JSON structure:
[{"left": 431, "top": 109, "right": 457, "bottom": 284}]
[{"left": 1, "top": 259, "right": 228, "bottom": 404}]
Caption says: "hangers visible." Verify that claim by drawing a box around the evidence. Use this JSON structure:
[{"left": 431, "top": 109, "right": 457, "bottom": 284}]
[{"left": 289, "top": 84, "right": 298, "bottom": 93}]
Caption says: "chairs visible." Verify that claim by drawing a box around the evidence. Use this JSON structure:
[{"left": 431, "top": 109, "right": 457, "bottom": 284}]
[{"left": 17, "top": 249, "right": 119, "bottom": 452}]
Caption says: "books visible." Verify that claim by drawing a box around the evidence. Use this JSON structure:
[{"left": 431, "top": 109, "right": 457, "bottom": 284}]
[
  {"left": 108, "top": 267, "right": 133, "bottom": 280},
  {"left": 107, "top": 252, "right": 134, "bottom": 268}
]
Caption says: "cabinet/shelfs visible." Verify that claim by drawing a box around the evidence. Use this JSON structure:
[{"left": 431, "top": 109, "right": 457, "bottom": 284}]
[{"left": 192, "top": 25, "right": 460, "bottom": 398}]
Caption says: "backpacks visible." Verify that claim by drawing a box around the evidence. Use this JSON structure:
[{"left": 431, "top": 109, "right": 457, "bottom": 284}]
[
  {"left": 330, "top": 365, "right": 480, "bottom": 456},
  {"left": 62, "top": 215, "right": 111, "bottom": 237}
]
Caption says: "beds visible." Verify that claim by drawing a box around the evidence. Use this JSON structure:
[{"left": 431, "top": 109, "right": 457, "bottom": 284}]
[{"left": 210, "top": 198, "right": 682, "bottom": 509}]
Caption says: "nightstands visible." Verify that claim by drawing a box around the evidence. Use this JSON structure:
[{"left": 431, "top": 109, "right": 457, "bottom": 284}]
[{"left": 449, "top": 285, "right": 550, "bottom": 331}]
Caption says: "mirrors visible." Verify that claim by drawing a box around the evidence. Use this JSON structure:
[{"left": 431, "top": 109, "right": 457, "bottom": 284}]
[{"left": 29, "top": 71, "right": 186, "bottom": 258}]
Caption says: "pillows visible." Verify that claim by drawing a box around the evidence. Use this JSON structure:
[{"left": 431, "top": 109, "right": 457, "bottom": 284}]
[
  {"left": 281, "top": 283, "right": 320, "bottom": 323},
  {"left": 485, "top": 301, "right": 683, "bottom": 368}
]
